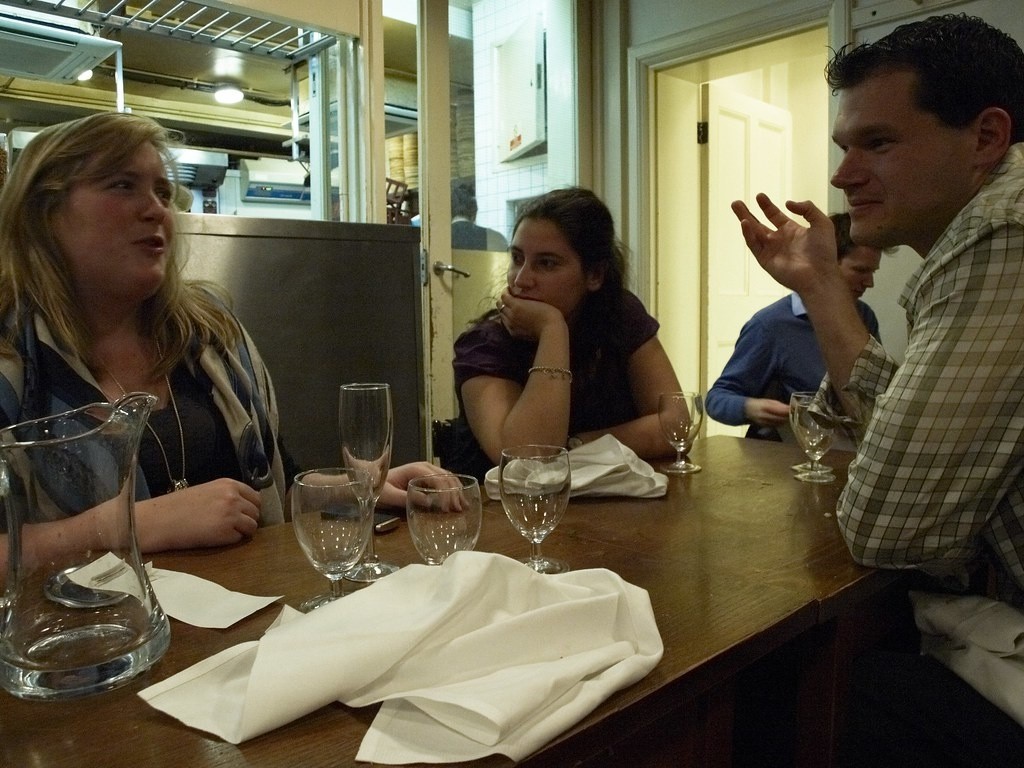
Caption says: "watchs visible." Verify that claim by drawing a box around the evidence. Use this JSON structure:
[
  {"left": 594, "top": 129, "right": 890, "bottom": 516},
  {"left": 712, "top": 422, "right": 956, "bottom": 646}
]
[{"left": 566, "top": 434, "right": 584, "bottom": 452}]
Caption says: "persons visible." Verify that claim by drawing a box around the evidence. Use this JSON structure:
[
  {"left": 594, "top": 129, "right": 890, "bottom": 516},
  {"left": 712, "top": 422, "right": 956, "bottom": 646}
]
[
  {"left": 451, "top": 186, "right": 690, "bottom": 486},
  {"left": 0, "top": 112, "right": 471, "bottom": 591},
  {"left": 731, "top": 11, "right": 1024, "bottom": 768},
  {"left": 451, "top": 187, "right": 509, "bottom": 252},
  {"left": 704, "top": 212, "right": 883, "bottom": 443}
]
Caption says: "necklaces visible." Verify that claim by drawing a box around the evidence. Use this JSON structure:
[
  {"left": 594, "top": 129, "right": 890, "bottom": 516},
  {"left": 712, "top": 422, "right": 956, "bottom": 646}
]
[{"left": 94, "top": 329, "right": 189, "bottom": 495}]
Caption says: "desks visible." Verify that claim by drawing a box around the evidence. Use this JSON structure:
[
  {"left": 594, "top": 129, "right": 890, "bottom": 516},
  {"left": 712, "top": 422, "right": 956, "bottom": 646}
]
[{"left": 0, "top": 438, "right": 910, "bottom": 768}]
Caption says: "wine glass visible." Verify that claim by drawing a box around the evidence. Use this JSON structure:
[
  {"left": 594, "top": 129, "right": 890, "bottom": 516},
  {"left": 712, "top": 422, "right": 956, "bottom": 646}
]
[
  {"left": 788, "top": 390, "right": 836, "bottom": 483},
  {"left": 290, "top": 467, "right": 376, "bottom": 612},
  {"left": 499, "top": 445, "right": 570, "bottom": 575},
  {"left": 339, "top": 383, "right": 400, "bottom": 583},
  {"left": 658, "top": 391, "right": 705, "bottom": 474}
]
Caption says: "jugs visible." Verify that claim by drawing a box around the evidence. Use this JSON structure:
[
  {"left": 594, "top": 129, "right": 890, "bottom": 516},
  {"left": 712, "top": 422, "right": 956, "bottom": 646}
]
[{"left": 0, "top": 392, "right": 172, "bottom": 702}]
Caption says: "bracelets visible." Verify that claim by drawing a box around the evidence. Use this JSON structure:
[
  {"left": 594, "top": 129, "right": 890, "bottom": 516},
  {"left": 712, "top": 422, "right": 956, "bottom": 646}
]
[{"left": 528, "top": 366, "right": 572, "bottom": 383}]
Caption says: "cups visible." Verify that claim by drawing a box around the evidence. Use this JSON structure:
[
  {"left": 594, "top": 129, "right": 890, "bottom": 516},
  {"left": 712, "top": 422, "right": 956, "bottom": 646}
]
[{"left": 407, "top": 472, "right": 481, "bottom": 565}]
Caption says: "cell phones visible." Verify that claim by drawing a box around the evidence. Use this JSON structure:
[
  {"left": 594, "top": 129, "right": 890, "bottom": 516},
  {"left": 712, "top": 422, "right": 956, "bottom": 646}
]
[{"left": 320, "top": 507, "right": 402, "bottom": 532}]
